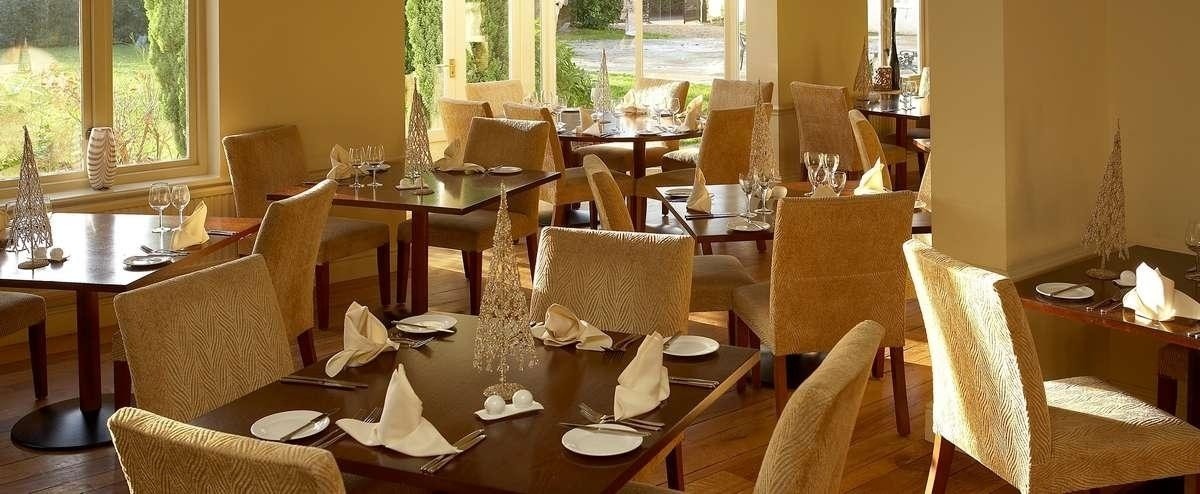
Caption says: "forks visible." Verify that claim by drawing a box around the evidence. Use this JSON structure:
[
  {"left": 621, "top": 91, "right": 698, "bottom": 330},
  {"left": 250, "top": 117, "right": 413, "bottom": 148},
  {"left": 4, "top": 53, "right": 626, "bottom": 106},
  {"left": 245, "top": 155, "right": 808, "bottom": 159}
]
[
  {"left": 581, "top": 409, "right": 659, "bottom": 431},
  {"left": 139, "top": 245, "right": 191, "bottom": 256},
  {"left": 615, "top": 335, "right": 642, "bottom": 352},
  {"left": 579, "top": 403, "right": 659, "bottom": 427},
  {"left": 316, "top": 406, "right": 383, "bottom": 450},
  {"left": 599, "top": 334, "right": 637, "bottom": 352},
  {"left": 1085, "top": 289, "right": 1126, "bottom": 310},
  {"left": 387, "top": 337, "right": 434, "bottom": 348}
]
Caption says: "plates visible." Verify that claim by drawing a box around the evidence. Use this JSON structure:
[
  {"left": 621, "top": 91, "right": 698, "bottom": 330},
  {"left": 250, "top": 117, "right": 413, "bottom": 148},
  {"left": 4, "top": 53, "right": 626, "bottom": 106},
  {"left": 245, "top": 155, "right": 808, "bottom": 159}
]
[
  {"left": 488, "top": 167, "right": 522, "bottom": 174},
  {"left": 636, "top": 129, "right": 661, "bottom": 135},
  {"left": 250, "top": 410, "right": 331, "bottom": 441},
  {"left": 472, "top": 401, "right": 545, "bottom": 421},
  {"left": 663, "top": 336, "right": 721, "bottom": 357},
  {"left": 393, "top": 183, "right": 429, "bottom": 190},
  {"left": 27, "top": 254, "right": 70, "bottom": 262},
  {"left": 561, "top": 424, "right": 644, "bottom": 457},
  {"left": 1035, "top": 281, "right": 1095, "bottom": 301},
  {"left": 122, "top": 255, "right": 172, "bottom": 267},
  {"left": 727, "top": 219, "right": 771, "bottom": 232},
  {"left": 663, "top": 187, "right": 713, "bottom": 199},
  {"left": 396, "top": 315, "right": 458, "bottom": 334},
  {"left": 359, "top": 164, "right": 392, "bottom": 172}
]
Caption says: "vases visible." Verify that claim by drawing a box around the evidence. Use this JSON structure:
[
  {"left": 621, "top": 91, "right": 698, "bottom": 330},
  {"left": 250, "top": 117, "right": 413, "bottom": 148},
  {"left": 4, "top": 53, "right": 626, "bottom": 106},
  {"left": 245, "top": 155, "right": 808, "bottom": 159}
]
[
  {"left": 888, "top": 6, "right": 900, "bottom": 97},
  {"left": 88, "top": 127, "right": 118, "bottom": 189}
]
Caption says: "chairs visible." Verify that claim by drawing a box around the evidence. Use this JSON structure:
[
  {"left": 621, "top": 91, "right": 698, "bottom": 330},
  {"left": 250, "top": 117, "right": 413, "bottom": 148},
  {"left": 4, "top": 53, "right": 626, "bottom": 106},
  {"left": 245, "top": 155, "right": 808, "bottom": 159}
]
[
  {"left": 111, "top": 255, "right": 295, "bottom": 424},
  {"left": 0, "top": 290, "right": 49, "bottom": 401},
  {"left": 879, "top": 67, "right": 932, "bottom": 181},
  {"left": 251, "top": 180, "right": 338, "bottom": 367},
  {"left": 105, "top": 402, "right": 346, "bottom": 493},
  {"left": 438, "top": 96, "right": 494, "bottom": 163},
  {"left": 582, "top": 153, "right": 760, "bottom": 386},
  {"left": 789, "top": 81, "right": 864, "bottom": 182},
  {"left": 661, "top": 79, "right": 774, "bottom": 216},
  {"left": 728, "top": 190, "right": 916, "bottom": 437},
  {"left": 901, "top": 237, "right": 1200, "bottom": 493},
  {"left": 529, "top": 226, "right": 695, "bottom": 494},
  {"left": 628, "top": 76, "right": 690, "bottom": 117},
  {"left": 396, "top": 116, "right": 552, "bottom": 315},
  {"left": 1155, "top": 340, "right": 1190, "bottom": 419},
  {"left": 634, "top": 106, "right": 756, "bottom": 233},
  {"left": 847, "top": 110, "right": 892, "bottom": 192},
  {"left": 464, "top": 78, "right": 524, "bottom": 118},
  {"left": 503, "top": 102, "right": 636, "bottom": 246},
  {"left": 617, "top": 319, "right": 886, "bottom": 493}
]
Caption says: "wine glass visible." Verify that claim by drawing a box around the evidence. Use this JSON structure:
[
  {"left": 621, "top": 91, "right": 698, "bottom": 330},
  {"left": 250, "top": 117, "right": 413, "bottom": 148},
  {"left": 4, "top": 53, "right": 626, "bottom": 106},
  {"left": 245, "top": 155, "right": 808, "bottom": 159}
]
[
  {"left": 755, "top": 168, "right": 775, "bottom": 215},
  {"left": 652, "top": 97, "right": 666, "bottom": 130},
  {"left": 828, "top": 171, "right": 847, "bottom": 198},
  {"left": 557, "top": 96, "right": 566, "bottom": 125},
  {"left": 739, "top": 172, "right": 758, "bottom": 217},
  {"left": 808, "top": 166, "right": 827, "bottom": 196},
  {"left": 822, "top": 154, "right": 840, "bottom": 186},
  {"left": 148, "top": 182, "right": 171, "bottom": 233},
  {"left": 171, "top": 184, "right": 191, "bottom": 232},
  {"left": 803, "top": 152, "right": 823, "bottom": 197},
  {"left": 551, "top": 96, "right": 562, "bottom": 130},
  {"left": 365, "top": 144, "right": 385, "bottom": 187},
  {"left": 666, "top": 98, "right": 680, "bottom": 128},
  {"left": 348, "top": 147, "right": 366, "bottom": 188}
]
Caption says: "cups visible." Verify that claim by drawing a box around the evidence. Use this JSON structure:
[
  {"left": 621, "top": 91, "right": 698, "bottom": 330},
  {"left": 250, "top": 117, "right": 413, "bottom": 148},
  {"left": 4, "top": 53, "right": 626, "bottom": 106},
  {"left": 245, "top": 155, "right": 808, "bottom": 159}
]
[
  {"left": 1184, "top": 223, "right": 1200, "bottom": 281},
  {"left": 905, "top": 81, "right": 917, "bottom": 109},
  {"left": 899, "top": 84, "right": 911, "bottom": 110}
]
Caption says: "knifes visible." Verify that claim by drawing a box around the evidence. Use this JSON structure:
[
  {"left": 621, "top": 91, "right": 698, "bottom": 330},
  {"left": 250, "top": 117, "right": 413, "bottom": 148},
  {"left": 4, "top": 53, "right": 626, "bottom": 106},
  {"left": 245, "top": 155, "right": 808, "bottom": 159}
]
[
  {"left": 279, "top": 378, "right": 356, "bottom": 390},
  {"left": 559, "top": 422, "right": 652, "bottom": 438},
  {"left": 684, "top": 211, "right": 737, "bottom": 220},
  {"left": 420, "top": 429, "right": 485, "bottom": 471},
  {"left": 279, "top": 408, "right": 341, "bottom": 443},
  {"left": 743, "top": 219, "right": 764, "bottom": 231},
  {"left": 1050, "top": 283, "right": 1090, "bottom": 297},
  {"left": 427, "top": 434, "right": 487, "bottom": 475},
  {"left": 288, "top": 375, "right": 369, "bottom": 388},
  {"left": 391, "top": 320, "right": 456, "bottom": 334}
]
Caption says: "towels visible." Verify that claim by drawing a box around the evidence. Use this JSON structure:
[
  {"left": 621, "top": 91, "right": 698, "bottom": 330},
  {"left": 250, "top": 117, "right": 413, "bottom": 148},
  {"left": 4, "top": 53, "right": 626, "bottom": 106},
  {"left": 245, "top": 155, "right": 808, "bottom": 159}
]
[
  {"left": 614, "top": 331, "right": 671, "bottom": 422},
  {"left": 325, "top": 301, "right": 401, "bottom": 379},
  {"left": 326, "top": 145, "right": 364, "bottom": 180},
  {"left": 531, "top": 303, "right": 613, "bottom": 353},
  {"left": 433, "top": 139, "right": 487, "bottom": 175},
  {"left": 688, "top": 164, "right": 711, "bottom": 218},
  {"left": 855, "top": 157, "right": 885, "bottom": 196},
  {"left": 623, "top": 90, "right": 648, "bottom": 117},
  {"left": 1123, "top": 262, "right": 1200, "bottom": 323},
  {"left": 571, "top": 106, "right": 601, "bottom": 138},
  {"left": 170, "top": 198, "right": 210, "bottom": 253},
  {"left": 0, "top": 208, "right": 11, "bottom": 235},
  {"left": 676, "top": 96, "right": 703, "bottom": 135},
  {"left": 342, "top": 365, "right": 464, "bottom": 458}
]
